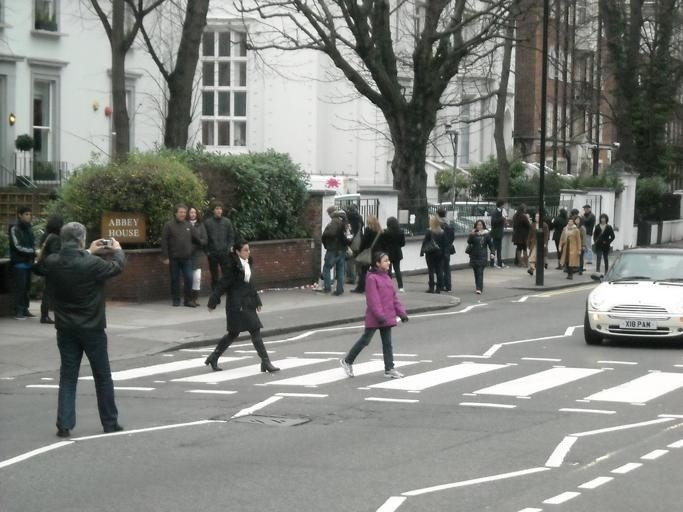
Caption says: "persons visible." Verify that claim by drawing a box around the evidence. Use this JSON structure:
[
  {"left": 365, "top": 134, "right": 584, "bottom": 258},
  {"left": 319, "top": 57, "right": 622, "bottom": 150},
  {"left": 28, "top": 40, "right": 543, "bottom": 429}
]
[
  {"left": 31, "top": 215, "right": 65, "bottom": 324},
  {"left": 205, "top": 239, "right": 279, "bottom": 373},
  {"left": 160, "top": 204, "right": 203, "bottom": 308},
  {"left": 340, "top": 250, "right": 408, "bottom": 380},
  {"left": 320, "top": 199, "right": 614, "bottom": 297},
  {"left": 202, "top": 200, "right": 234, "bottom": 303},
  {"left": 186, "top": 205, "right": 209, "bottom": 306},
  {"left": 9, "top": 207, "right": 36, "bottom": 319},
  {"left": 42, "top": 221, "right": 126, "bottom": 437}
]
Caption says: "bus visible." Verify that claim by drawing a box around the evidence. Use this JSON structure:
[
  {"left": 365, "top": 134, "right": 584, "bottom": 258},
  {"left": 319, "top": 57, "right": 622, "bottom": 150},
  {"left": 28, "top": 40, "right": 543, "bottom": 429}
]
[{"left": 437, "top": 201, "right": 497, "bottom": 229}]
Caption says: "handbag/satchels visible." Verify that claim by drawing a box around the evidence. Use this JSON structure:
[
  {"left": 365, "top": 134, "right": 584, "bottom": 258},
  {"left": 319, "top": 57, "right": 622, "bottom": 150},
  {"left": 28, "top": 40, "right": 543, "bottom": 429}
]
[
  {"left": 591, "top": 244, "right": 596, "bottom": 252},
  {"left": 345, "top": 247, "right": 354, "bottom": 260},
  {"left": 354, "top": 248, "right": 371, "bottom": 265},
  {"left": 33, "top": 235, "right": 53, "bottom": 276},
  {"left": 424, "top": 231, "right": 439, "bottom": 253},
  {"left": 450, "top": 246, "right": 455, "bottom": 255},
  {"left": 465, "top": 243, "right": 473, "bottom": 254}
]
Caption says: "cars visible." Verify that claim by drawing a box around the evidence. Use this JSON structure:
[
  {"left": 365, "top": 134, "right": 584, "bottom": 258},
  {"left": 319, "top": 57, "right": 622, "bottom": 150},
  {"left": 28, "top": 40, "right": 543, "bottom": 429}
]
[{"left": 582, "top": 248, "right": 683, "bottom": 346}]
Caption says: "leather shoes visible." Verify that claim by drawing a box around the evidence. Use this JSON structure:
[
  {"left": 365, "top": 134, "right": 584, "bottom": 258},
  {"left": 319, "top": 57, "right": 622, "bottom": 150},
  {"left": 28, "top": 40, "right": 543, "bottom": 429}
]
[
  {"left": 55, "top": 428, "right": 69, "bottom": 435},
  {"left": 103, "top": 422, "right": 122, "bottom": 432}
]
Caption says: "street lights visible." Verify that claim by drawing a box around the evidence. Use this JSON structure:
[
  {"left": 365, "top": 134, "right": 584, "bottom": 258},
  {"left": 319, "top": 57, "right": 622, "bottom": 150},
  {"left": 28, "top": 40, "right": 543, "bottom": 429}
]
[{"left": 444, "top": 121, "right": 458, "bottom": 211}]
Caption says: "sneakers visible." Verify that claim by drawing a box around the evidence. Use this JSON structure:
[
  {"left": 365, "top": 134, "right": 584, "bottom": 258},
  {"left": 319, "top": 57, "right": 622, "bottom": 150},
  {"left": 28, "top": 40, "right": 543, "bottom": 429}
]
[
  {"left": 340, "top": 359, "right": 354, "bottom": 378},
  {"left": 184, "top": 302, "right": 196, "bottom": 307},
  {"left": 325, "top": 287, "right": 363, "bottom": 295},
  {"left": 476, "top": 290, "right": 481, "bottom": 294},
  {"left": 425, "top": 287, "right": 451, "bottom": 293},
  {"left": 25, "top": 311, "right": 35, "bottom": 318},
  {"left": 15, "top": 312, "right": 26, "bottom": 320},
  {"left": 528, "top": 268, "right": 533, "bottom": 275},
  {"left": 384, "top": 369, "right": 404, "bottom": 379},
  {"left": 173, "top": 298, "right": 181, "bottom": 306},
  {"left": 400, "top": 289, "right": 406, "bottom": 293}
]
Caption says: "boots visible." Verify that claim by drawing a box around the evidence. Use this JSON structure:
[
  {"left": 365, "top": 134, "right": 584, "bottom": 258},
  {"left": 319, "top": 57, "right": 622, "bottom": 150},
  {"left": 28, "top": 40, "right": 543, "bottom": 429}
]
[
  {"left": 312, "top": 279, "right": 324, "bottom": 291},
  {"left": 40, "top": 304, "right": 55, "bottom": 323},
  {"left": 193, "top": 290, "right": 201, "bottom": 306},
  {"left": 206, "top": 335, "right": 233, "bottom": 371},
  {"left": 252, "top": 337, "right": 280, "bottom": 372}
]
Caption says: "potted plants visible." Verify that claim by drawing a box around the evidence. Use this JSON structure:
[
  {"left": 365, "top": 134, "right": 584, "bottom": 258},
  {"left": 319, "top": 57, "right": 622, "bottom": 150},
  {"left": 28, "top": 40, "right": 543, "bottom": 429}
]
[{"left": 13, "top": 135, "right": 35, "bottom": 187}]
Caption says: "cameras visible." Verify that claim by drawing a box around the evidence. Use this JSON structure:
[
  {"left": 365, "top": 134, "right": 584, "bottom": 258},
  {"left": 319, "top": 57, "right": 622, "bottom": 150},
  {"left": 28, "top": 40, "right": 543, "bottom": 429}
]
[{"left": 99, "top": 240, "right": 111, "bottom": 248}]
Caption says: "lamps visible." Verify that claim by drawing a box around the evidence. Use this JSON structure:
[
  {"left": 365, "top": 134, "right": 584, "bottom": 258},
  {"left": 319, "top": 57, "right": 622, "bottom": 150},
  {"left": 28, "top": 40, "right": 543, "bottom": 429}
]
[{"left": 8, "top": 113, "right": 15, "bottom": 126}]
